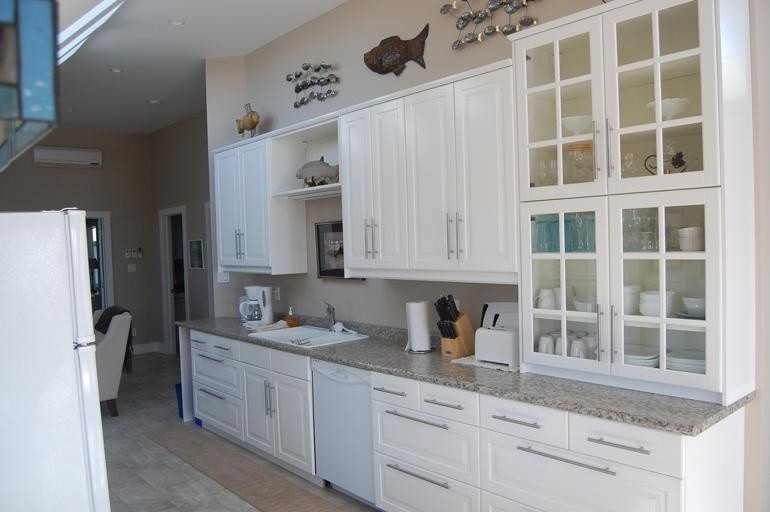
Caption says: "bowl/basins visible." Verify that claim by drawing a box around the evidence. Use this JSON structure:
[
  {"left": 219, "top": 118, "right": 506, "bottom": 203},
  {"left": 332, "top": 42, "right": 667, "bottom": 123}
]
[
  {"left": 639, "top": 291, "right": 675, "bottom": 315},
  {"left": 573, "top": 295, "right": 595, "bottom": 312},
  {"left": 652, "top": 99, "right": 690, "bottom": 118},
  {"left": 679, "top": 237, "right": 703, "bottom": 251},
  {"left": 681, "top": 295, "right": 704, "bottom": 314},
  {"left": 677, "top": 227, "right": 702, "bottom": 239},
  {"left": 563, "top": 116, "right": 590, "bottom": 133}
]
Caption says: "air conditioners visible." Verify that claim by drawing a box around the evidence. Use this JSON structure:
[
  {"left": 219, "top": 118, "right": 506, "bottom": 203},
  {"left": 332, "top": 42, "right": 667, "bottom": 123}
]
[{"left": 32, "top": 148, "right": 102, "bottom": 167}]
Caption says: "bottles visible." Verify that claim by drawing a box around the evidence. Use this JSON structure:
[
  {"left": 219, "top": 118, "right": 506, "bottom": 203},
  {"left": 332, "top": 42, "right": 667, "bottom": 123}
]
[{"left": 565, "top": 145, "right": 596, "bottom": 185}]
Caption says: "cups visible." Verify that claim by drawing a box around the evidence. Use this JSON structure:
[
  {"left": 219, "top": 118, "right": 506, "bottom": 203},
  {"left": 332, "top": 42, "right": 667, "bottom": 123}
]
[
  {"left": 586, "top": 336, "right": 596, "bottom": 359},
  {"left": 557, "top": 287, "right": 571, "bottom": 310},
  {"left": 568, "top": 219, "right": 596, "bottom": 252},
  {"left": 625, "top": 207, "right": 657, "bottom": 251},
  {"left": 538, "top": 218, "right": 561, "bottom": 251},
  {"left": 534, "top": 158, "right": 559, "bottom": 187},
  {"left": 551, "top": 325, "right": 597, "bottom": 340},
  {"left": 620, "top": 151, "right": 637, "bottom": 177},
  {"left": 534, "top": 289, "right": 554, "bottom": 309},
  {"left": 624, "top": 286, "right": 641, "bottom": 313},
  {"left": 553, "top": 335, "right": 569, "bottom": 354},
  {"left": 571, "top": 339, "right": 585, "bottom": 357},
  {"left": 538, "top": 335, "right": 553, "bottom": 353}
]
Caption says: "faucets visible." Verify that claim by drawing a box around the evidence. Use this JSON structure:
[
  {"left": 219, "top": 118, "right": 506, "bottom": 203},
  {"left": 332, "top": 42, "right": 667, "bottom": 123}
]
[{"left": 322, "top": 296, "right": 336, "bottom": 332}]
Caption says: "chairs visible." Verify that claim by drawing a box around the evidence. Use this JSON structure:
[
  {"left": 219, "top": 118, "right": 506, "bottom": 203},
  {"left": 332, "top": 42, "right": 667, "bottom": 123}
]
[{"left": 93, "top": 310, "right": 132, "bottom": 416}]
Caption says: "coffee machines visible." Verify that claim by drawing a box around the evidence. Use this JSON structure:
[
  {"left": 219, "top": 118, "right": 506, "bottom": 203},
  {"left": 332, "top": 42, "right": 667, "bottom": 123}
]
[{"left": 239, "top": 286, "right": 273, "bottom": 325}]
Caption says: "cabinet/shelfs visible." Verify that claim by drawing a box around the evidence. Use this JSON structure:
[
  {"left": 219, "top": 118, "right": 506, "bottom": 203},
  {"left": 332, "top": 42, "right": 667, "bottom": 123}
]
[
  {"left": 241, "top": 342, "right": 314, "bottom": 478},
  {"left": 337, "top": 90, "right": 404, "bottom": 282},
  {"left": 212, "top": 133, "right": 307, "bottom": 275},
  {"left": 369, "top": 371, "right": 481, "bottom": 512},
  {"left": 508, "top": 1, "right": 757, "bottom": 407},
  {"left": 405, "top": 59, "right": 521, "bottom": 285},
  {"left": 480, "top": 393, "right": 746, "bottom": 512},
  {"left": 189, "top": 329, "right": 244, "bottom": 445}
]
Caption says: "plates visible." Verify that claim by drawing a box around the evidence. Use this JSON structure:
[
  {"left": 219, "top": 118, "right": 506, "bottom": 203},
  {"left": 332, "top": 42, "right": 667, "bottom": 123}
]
[
  {"left": 625, "top": 343, "right": 667, "bottom": 368},
  {"left": 680, "top": 311, "right": 704, "bottom": 319},
  {"left": 667, "top": 351, "right": 706, "bottom": 374}
]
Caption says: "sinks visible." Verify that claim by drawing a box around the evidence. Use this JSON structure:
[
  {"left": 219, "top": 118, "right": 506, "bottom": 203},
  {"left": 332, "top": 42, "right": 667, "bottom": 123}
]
[{"left": 249, "top": 325, "right": 369, "bottom": 347}]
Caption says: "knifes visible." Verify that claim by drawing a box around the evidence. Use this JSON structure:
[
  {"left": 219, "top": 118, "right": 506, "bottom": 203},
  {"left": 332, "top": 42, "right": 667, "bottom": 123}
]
[{"left": 434, "top": 294, "right": 458, "bottom": 336}]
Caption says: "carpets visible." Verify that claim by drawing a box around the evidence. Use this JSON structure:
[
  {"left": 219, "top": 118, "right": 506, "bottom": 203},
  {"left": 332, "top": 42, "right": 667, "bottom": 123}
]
[{"left": 98, "top": 351, "right": 262, "bottom": 512}]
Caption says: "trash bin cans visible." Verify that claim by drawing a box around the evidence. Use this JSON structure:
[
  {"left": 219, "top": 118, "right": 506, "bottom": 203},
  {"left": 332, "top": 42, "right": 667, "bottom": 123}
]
[{"left": 176, "top": 384, "right": 184, "bottom": 418}]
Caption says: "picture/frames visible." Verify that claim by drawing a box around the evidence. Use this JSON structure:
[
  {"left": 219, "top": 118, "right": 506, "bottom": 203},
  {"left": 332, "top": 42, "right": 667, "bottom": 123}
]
[
  {"left": 315, "top": 221, "right": 366, "bottom": 281},
  {"left": 188, "top": 239, "right": 205, "bottom": 270}
]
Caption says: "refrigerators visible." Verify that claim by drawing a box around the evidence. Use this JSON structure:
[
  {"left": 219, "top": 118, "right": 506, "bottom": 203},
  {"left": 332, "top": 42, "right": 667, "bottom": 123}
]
[{"left": 0, "top": 208, "right": 112, "bottom": 512}]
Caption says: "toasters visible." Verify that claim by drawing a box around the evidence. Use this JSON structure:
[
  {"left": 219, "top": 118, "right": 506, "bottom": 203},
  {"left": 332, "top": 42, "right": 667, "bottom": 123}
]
[{"left": 472, "top": 323, "right": 518, "bottom": 369}]
